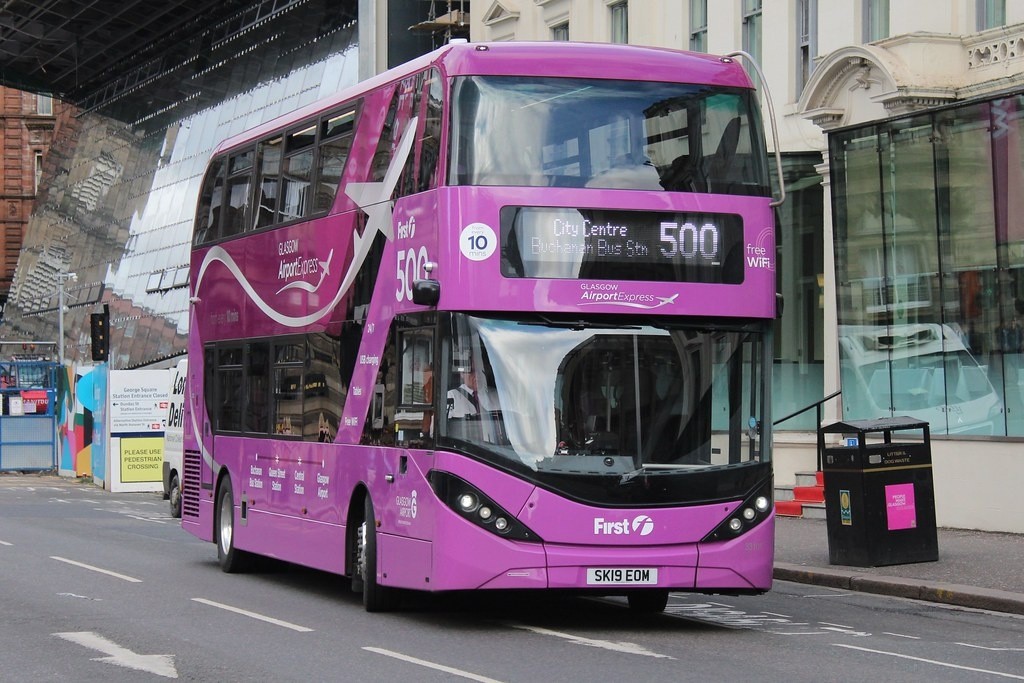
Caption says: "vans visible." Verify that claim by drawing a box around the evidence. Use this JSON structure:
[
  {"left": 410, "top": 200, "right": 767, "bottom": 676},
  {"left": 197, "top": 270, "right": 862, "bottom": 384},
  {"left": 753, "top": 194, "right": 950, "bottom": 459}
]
[
  {"left": 160, "top": 359, "right": 188, "bottom": 518},
  {"left": 837, "top": 319, "right": 1006, "bottom": 437}
]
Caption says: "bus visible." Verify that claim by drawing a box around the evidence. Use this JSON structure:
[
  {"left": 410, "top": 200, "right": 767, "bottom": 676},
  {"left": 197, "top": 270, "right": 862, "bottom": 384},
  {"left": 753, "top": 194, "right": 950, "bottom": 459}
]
[{"left": 181, "top": 38, "right": 788, "bottom": 613}]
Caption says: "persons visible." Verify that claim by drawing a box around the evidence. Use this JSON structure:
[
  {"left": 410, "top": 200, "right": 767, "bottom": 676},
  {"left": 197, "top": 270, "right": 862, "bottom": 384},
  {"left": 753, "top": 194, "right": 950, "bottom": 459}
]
[{"left": 429, "top": 357, "right": 508, "bottom": 444}]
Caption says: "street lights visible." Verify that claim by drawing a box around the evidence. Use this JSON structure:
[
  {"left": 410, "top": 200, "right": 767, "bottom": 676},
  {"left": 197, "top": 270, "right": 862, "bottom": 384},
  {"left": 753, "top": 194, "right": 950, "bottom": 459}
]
[{"left": 47, "top": 270, "right": 79, "bottom": 368}]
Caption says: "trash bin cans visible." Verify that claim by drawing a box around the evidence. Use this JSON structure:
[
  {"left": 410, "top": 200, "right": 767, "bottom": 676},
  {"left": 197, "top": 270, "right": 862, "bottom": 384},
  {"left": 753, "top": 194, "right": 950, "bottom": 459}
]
[{"left": 819, "top": 417, "right": 939, "bottom": 567}]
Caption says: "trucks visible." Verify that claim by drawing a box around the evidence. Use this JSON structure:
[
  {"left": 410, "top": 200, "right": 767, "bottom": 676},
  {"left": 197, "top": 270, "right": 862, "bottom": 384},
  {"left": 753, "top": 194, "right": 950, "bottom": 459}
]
[{"left": 0, "top": 343, "right": 59, "bottom": 414}]
